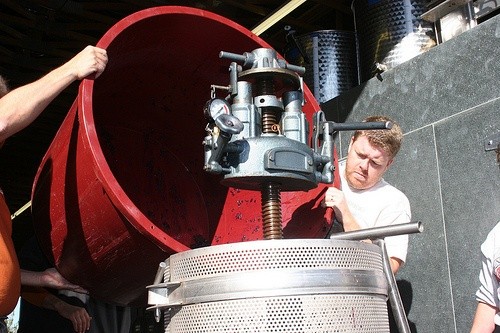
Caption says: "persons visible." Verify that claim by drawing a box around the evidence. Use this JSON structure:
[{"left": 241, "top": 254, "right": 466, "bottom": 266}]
[
  {"left": 0, "top": 45, "right": 110, "bottom": 333},
  {"left": 470, "top": 219, "right": 500, "bottom": 333},
  {"left": 320, "top": 116, "right": 412, "bottom": 276},
  {"left": 16, "top": 249, "right": 94, "bottom": 333}
]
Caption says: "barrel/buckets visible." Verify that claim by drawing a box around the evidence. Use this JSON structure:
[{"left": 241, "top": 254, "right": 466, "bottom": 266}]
[{"left": 31, "top": 6, "right": 339, "bottom": 308}]
